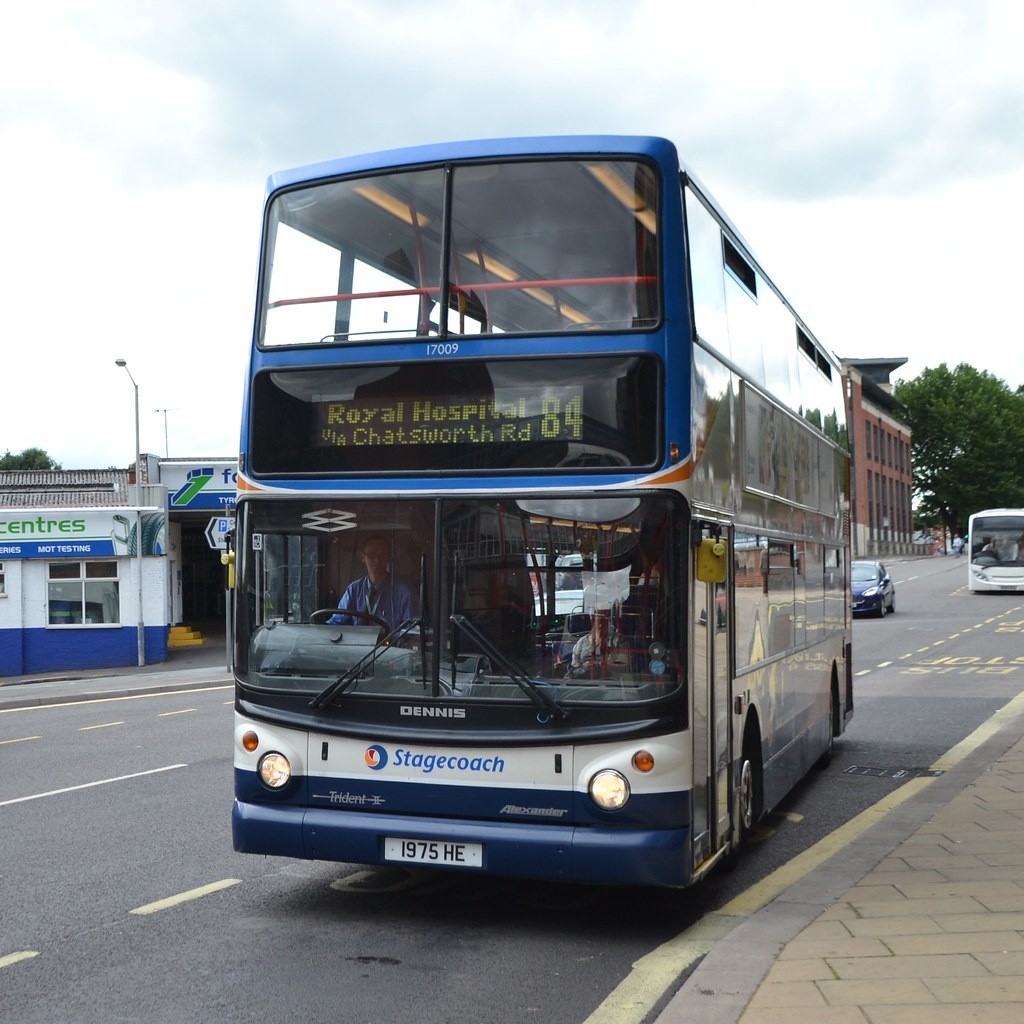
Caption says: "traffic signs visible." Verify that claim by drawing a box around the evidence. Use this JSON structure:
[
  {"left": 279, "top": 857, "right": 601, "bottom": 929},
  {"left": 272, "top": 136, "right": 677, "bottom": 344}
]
[{"left": 203, "top": 518, "right": 236, "bottom": 549}]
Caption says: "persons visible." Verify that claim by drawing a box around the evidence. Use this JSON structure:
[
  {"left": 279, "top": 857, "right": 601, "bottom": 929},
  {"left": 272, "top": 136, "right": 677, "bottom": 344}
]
[
  {"left": 450, "top": 501, "right": 681, "bottom": 673},
  {"left": 321, "top": 534, "right": 414, "bottom": 647},
  {"left": 953, "top": 533, "right": 962, "bottom": 558},
  {"left": 981, "top": 532, "right": 1023, "bottom": 560}
]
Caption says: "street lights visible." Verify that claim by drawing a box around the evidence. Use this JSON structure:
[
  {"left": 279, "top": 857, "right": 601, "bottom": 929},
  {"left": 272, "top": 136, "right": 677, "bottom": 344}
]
[
  {"left": 154, "top": 408, "right": 170, "bottom": 457},
  {"left": 114, "top": 357, "right": 146, "bottom": 667}
]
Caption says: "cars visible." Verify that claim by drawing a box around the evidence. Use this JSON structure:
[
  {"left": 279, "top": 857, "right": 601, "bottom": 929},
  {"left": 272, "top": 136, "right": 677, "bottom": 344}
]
[{"left": 850, "top": 556, "right": 898, "bottom": 620}]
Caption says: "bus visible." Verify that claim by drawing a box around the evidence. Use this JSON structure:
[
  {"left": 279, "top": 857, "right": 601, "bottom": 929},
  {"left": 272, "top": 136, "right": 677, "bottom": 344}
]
[
  {"left": 216, "top": 132, "right": 859, "bottom": 892},
  {"left": 963, "top": 507, "right": 1024, "bottom": 594}
]
